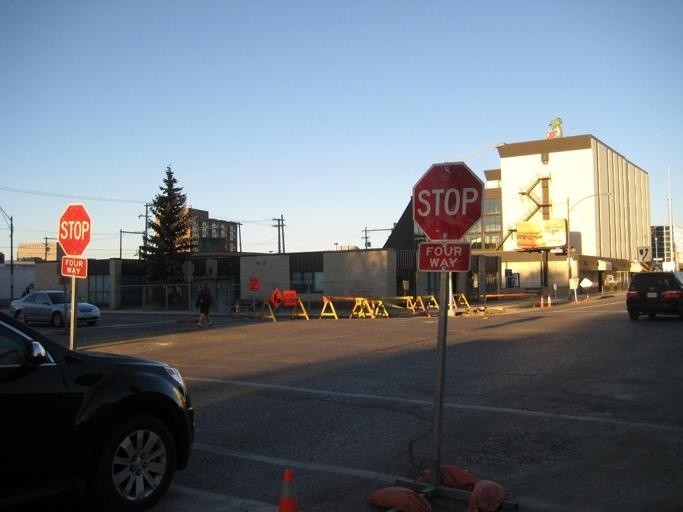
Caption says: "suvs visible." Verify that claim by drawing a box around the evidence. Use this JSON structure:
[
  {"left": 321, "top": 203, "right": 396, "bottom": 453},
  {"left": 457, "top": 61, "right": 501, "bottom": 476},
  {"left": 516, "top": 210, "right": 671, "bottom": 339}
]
[
  {"left": 626, "top": 269, "right": 683, "bottom": 320},
  {"left": 0, "top": 313, "right": 196, "bottom": 508}
]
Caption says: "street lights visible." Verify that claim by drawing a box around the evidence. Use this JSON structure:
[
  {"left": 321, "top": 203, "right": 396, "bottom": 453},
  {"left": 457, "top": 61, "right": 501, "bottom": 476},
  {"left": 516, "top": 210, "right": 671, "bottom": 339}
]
[{"left": 566, "top": 192, "right": 612, "bottom": 302}]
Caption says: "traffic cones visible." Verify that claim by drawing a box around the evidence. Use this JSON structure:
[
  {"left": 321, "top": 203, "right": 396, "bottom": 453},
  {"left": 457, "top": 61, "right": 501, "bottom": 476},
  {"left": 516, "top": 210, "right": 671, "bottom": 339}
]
[
  {"left": 231, "top": 300, "right": 241, "bottom": 319},
  {"left": 278, "top": 466, "right": 299, "bottom": 511}
]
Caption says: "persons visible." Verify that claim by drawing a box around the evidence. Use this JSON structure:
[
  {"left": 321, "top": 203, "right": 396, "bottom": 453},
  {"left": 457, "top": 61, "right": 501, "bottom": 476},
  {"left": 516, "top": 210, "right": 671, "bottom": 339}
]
[
  {"left": 22, "top": 285, "right": 30, "bottom": 299},
  {"left": 195, "top": 279, "right": 214, "bottom": 328}
]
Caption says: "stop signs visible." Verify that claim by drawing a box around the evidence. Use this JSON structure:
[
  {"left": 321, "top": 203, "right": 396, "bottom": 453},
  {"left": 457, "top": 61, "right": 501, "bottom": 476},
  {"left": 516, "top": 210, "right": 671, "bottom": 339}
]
[
  {"left": 411, "top": 162, "right": 486, "bottom": 242},
  {"left": 58, "top": 203, "right": 93, "bottom": 256}
]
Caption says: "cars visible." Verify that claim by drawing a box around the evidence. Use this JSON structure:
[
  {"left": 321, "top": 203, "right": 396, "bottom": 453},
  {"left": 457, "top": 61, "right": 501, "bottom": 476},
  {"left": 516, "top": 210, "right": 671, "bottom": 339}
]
[{"left": 11, "top": 290, "right": 100, "bottom": 328}]
